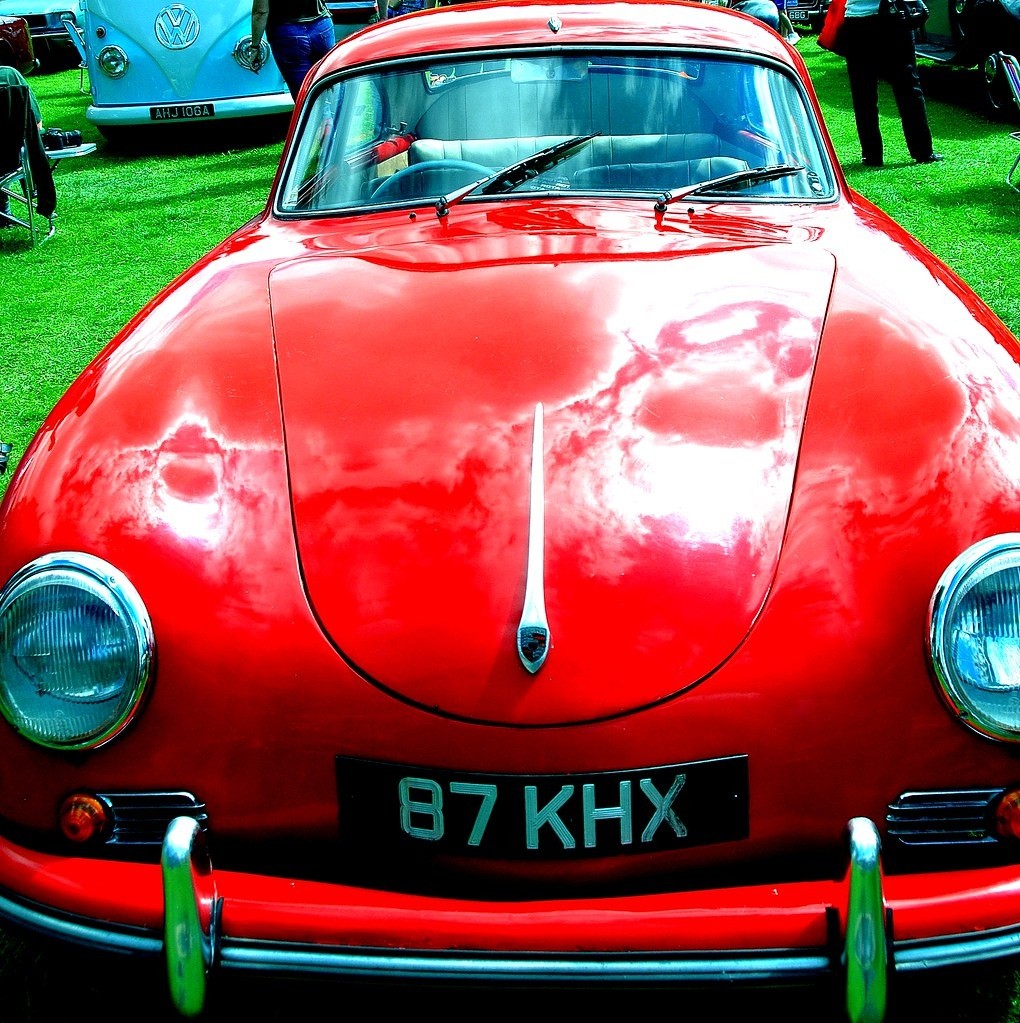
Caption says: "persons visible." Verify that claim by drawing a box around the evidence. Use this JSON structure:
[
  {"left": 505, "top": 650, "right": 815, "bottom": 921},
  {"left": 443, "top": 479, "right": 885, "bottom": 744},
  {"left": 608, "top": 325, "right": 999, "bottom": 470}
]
[
  {"left": 774, "top": 0, "right": 802, "bottom": 46},
  {"left": 376, "top": 0, "right": 437, "bottom": 22},
  {"left": 248, "top": 0, "right": 335, "bottom": 104},
  {"left": 1, "top": 65, "right": 44, "bottom": 226},
  {"left": 816, "top": 0, "right": 944, "bottom": 167}
]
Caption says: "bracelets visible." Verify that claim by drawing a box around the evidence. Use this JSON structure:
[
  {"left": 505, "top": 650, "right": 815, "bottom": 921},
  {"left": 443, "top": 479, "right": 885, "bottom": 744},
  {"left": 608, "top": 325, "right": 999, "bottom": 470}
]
[{"left": 250, "top": 45, "right": 260, "bottom": 49}]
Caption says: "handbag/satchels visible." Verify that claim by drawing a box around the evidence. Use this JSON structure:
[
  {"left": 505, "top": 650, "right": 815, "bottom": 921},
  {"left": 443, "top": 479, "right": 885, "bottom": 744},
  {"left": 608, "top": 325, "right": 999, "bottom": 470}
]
[{"left": 878, "top": 0, "right": 930, "bottom": 32}]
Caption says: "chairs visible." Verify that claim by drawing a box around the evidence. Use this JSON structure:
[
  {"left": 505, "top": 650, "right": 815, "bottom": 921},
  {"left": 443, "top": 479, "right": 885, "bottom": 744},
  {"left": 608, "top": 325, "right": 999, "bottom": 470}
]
[
  {"left": 998, "top": 50, "right": 1020, "bottom": 195},
  {"left": 576, "top": 157, "right": 754, "bottom": 198},
  {"left": 0, "top": 85, "right": 57, "bottom": 250},
  {"left": 358, "top": 167, "right": 534, "bottom": 202}
]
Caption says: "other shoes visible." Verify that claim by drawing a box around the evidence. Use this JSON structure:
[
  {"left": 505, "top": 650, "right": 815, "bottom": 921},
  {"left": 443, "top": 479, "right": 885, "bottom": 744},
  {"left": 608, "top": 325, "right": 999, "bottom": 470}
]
[
  {"left": 916, "top": 153, "right": 944, "bottom": 163},
  {"left": 788, "top": 32, "right": 801, "bottom": 45},
  {"left": 862, "top": 159, "right": 883, "bottom": 167}
]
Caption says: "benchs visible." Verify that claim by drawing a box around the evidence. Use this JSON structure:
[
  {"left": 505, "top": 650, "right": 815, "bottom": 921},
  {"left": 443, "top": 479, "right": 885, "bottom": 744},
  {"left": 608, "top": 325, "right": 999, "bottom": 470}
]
[{"left": 408, "top": 133, "right": 721, "bottom": 188}]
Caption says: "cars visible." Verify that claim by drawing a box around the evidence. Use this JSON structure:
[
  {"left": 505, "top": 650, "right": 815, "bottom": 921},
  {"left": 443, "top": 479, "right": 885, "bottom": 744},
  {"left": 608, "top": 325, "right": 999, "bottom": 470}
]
[
  {"left": 775, "top": 1, "right": 837, "bottom": 34},
  {"left": 1, "top": 0, "right": 1020, "bottom": 1022},
  {"left": 0, "top": 9, "right": 87, "bottom": 76}
]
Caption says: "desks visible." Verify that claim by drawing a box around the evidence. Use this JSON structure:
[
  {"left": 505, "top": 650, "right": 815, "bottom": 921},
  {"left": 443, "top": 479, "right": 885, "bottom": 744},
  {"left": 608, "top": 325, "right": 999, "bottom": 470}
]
[{"left": 20, "top": 143, "right": 99, "bottom": 200}]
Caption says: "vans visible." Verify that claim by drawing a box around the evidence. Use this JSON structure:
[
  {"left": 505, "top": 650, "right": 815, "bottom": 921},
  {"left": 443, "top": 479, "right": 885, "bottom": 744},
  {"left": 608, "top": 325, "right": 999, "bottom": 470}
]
[{"left": 61, "top": 0, "right": 298, "bottom": 150}]
